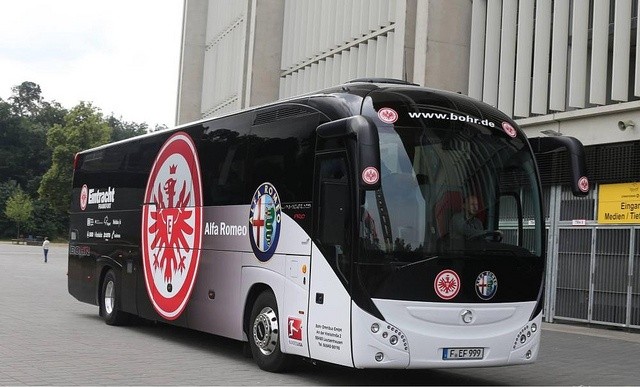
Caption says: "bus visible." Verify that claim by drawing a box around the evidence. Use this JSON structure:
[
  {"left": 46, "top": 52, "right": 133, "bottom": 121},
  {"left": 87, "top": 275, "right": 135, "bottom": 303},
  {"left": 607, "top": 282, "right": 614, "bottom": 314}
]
[{"left": 66, "top": 76, "right": 592, "bottom": 373}]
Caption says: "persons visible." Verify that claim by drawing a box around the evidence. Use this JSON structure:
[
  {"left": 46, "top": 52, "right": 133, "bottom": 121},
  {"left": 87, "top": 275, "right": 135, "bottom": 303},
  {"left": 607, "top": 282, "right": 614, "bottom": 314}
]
[
  {"left": 43, "top": 237, "right": 50, "bottom": 263},
  {"left": 451, "top": 194, "right": 488, "bottom": 253}
]
